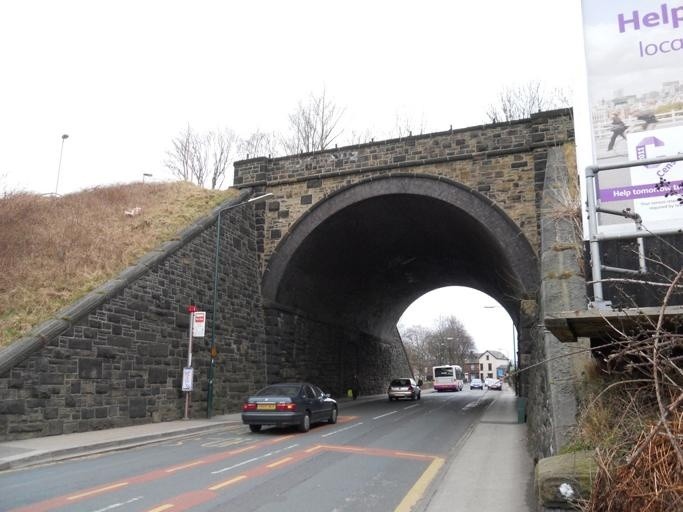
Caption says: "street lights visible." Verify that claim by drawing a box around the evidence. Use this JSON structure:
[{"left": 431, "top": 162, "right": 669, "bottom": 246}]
[
  {"left": 484, "top": 305, "right": 517, "bottom": 395},
  {"left": 205, "top": 192, "right": 281, "bottom": 414},
  {"left": 141, "top": 172, "right": 153, "bottom": 183},
  {"left": 53, "top": 132, "right": 70, "bottom": 196}
]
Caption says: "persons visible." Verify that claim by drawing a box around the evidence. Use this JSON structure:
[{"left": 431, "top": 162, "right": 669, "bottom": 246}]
[
  {"left": 606, "top": 109, "right": 658, "bottom": 152},
  {"left": 351, "top": 374, "right": 360, "bottom": 400}
]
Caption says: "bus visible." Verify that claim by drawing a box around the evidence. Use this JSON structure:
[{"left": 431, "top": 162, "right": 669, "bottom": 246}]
[{"left": 432, "top": 364, "right": 464, "bottom": 393}]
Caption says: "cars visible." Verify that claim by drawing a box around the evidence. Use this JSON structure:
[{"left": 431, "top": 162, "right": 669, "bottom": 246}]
[
  {"left": 238, "top": 382, "right": 338, "bottom": 433},
  {"left": 388, "top": 378, "right": 420, "bottom": 401},
  {"left": 488, "top": 380, "right": 501, "bottom": 391},
  {"left": 469, "top": 379, "right": 484, "bottom": 390},
  {"left": 485, "top": 378, "right": 493, "bottom": 386}
]
